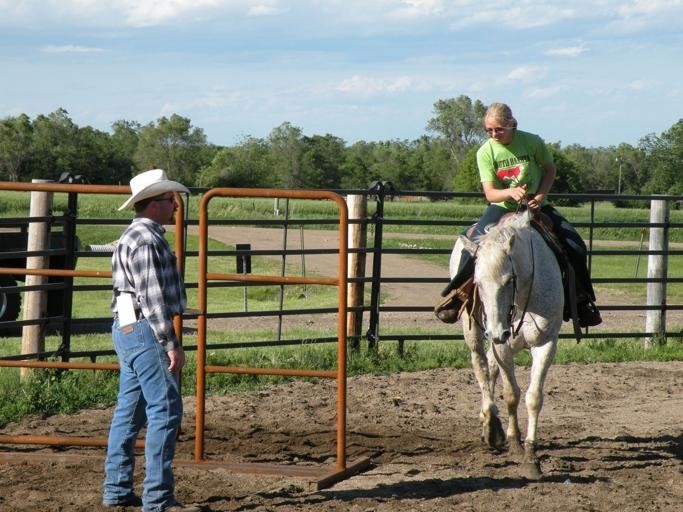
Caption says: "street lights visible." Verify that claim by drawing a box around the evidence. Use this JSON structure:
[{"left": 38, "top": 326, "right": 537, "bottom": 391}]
[{"left": 615, "top": 155, "right": 623, "bottom": 196}]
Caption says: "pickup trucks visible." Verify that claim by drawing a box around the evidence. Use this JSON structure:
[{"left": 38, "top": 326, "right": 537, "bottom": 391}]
[{"left": 0, "top": 229, "right": 81, "bottom": 325}]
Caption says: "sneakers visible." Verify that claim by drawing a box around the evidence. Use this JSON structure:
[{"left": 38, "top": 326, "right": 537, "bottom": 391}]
[
  {"left": 160, "top": 500, "right": 201, "bottom": 512},
  {"left": 103, "top": 494, "right": 143, "bottom": 507},
  {"left": 438, "top": 299, "right": 461, "bottom": 323},
  {"left": 577, "top": 303, "right": 602, "bottom": 327}
]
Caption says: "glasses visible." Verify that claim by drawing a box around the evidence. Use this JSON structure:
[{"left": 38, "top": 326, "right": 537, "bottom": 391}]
[{"left": 486, "top": 123, "right": 509, "bottom": 135}]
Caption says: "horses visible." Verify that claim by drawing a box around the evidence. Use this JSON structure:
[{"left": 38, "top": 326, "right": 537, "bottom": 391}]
[{"left": 445, "top": 207, "right": 568, "bottom": 481}]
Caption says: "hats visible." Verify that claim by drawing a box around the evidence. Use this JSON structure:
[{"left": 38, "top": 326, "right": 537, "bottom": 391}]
[{"left": 117, "top": 168, "right": 190, "bottom": 211}]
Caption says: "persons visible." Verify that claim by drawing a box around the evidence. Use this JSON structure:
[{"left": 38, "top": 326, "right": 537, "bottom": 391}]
[
  {"left": 436, "top": 101, "right": 602, "bottom": 326},
  {"left": 102, "top": 169, "right": 205, "bottom": 512}
]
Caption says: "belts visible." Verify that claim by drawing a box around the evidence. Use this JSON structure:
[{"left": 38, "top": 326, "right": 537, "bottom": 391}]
[{"left": 115, "top": 310, "right": 144, "bottom": 320}]
